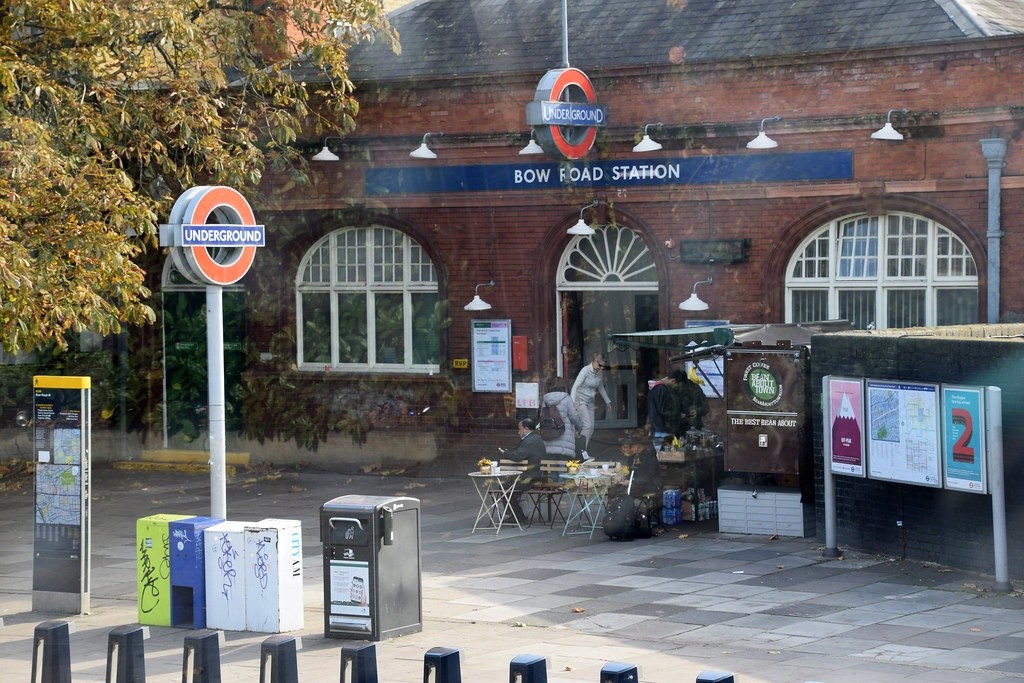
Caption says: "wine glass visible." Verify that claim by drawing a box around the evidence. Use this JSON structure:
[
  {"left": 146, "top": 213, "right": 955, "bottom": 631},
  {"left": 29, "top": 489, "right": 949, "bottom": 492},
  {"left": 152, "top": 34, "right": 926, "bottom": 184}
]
[{"left": 653, "top": 439, "right": 663, "bottom": 457}]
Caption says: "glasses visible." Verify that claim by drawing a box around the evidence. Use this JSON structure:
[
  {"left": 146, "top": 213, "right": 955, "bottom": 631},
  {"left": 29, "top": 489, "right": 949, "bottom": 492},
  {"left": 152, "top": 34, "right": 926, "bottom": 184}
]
[{"left": 597, "top": 360, "right": 606, "bottom": 369}]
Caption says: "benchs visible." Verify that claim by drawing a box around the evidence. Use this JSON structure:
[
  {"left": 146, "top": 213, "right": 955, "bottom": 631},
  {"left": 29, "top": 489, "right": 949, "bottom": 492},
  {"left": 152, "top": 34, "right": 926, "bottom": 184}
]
[{"left": 487, "top": 460, "right": 623, "bottom": 524}]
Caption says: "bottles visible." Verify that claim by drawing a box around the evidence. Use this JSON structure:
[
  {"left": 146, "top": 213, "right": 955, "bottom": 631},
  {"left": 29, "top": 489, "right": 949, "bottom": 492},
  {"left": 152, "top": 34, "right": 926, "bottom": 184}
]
[
  {"left": 665, "top": 426, "right": 725, "bottom": 458},
  {"left": 662, "top": 487, "right": 717, "bottom": 525}
]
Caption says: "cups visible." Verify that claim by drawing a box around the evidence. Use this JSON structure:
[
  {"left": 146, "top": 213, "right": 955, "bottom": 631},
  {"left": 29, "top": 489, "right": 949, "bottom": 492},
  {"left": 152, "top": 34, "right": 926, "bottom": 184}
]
[
  {"left": 602, "top": 465, "right": 609, "bottom": 473},
  {"left": 494, "top": 467, "right": 500, "bottom": 473},
  {"left": 590, "top": 469, "right": 598, "bottom": 476},
  {"left": 492, "top": 462, "right": 497, "bottom": 473}
]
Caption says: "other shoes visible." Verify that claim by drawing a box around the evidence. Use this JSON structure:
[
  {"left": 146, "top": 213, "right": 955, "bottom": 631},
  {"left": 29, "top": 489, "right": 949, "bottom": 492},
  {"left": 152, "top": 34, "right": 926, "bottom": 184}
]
[{"left": 505, "top": 514, "right": 526, "bottom": 523}]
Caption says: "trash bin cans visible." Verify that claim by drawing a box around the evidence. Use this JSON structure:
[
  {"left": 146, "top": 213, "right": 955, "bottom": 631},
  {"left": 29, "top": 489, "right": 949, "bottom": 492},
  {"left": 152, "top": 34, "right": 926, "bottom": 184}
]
[{"left": 320, "top": 494, "right": 422, "bottom": 641}]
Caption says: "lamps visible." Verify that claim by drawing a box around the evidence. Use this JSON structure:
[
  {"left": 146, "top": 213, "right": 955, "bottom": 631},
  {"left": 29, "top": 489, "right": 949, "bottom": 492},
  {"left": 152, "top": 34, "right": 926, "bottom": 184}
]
[
  {"left": 678, "top": 276, "right": 714, "bottom": 310},
  {"left": 519, "top": 130, "right": 544, "bottom": 154},
  {"left": 632, "top": 123, "right": 662, "bottom": 151},
  {"left": 313, "top": 136, "right": 355, "bottom": 161},
  {"left": 464, "top": 281, "right": 496, "bottom": 310},
  {"left": 746, "top": 116, "right": 782, "bottom": 149},
  {"left": 870, "top": 109, "right": 908, "bottom": 140},
  {"left": 410, "top": 132, "right": 443, "bottom": 158},
  {"left": 566, "top": 200, "right": 599, "bottom": 234}
]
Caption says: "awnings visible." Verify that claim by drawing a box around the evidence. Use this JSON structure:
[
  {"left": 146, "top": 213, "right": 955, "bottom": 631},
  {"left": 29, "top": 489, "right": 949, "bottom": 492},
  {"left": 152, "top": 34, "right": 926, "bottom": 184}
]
[{"left": 611, "top": 319, "right": 850, "bottom": 348}]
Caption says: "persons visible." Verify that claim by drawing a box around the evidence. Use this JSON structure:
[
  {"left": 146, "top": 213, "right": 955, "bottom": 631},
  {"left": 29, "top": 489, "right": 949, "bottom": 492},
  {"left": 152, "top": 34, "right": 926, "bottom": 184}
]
[
  {"left": 541, "top": 376, "right": 583, "bottom": 493},
  {"left": 498, "top": 417, "right": 547, "bottom": 525},
  {"left": 636, "top": 369, "right": 708, "bottom": 446},
  {"left": 570, "top": 352, "right": 612, "bottom": 459}
]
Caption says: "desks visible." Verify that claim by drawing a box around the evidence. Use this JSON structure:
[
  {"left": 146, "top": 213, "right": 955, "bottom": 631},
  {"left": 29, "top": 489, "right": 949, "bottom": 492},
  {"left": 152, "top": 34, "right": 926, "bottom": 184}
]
[
  {"left": 656, "top": 453, "right": 724, "bottom": 494},
  {"left": 468, "top": 470, "right": 523, "bottom": 534}
]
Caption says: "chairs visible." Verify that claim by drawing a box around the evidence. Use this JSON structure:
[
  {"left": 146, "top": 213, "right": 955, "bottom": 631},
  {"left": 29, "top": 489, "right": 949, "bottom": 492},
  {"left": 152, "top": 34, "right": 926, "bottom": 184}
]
[
  {"left": 487, "top": 460, "right": 528, "bottom": 527},
  {"left": 577, "top": 461, "right": 618, "bottom": 530},
  {"left": 527, "top": 462, "right": 571, "bottom": 527}
]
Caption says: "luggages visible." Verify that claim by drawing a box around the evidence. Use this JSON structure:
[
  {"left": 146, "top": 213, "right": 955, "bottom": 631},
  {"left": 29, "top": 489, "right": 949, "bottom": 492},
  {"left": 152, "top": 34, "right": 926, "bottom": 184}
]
[{"left": 603, "top": 466, "right": 639, "bottom": 542}]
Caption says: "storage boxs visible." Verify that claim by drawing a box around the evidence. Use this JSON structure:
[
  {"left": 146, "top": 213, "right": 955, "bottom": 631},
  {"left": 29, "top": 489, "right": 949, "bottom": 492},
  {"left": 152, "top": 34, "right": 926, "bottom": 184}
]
[{"left": 643, "top": 488, "right": 717, "bottom": 527}]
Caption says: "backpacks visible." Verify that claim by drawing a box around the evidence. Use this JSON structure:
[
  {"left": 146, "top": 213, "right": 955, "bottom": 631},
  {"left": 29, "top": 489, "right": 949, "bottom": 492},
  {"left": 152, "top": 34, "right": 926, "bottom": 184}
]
[{"left": 539, "top": 395, "right": 567, "bottom": 440}]
